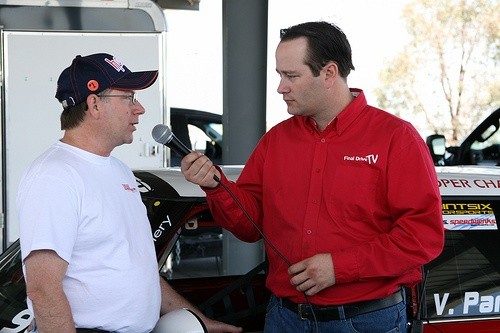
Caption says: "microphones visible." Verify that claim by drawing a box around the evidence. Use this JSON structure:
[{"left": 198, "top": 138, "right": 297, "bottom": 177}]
[{"left": 152, "top": 125, "right": 219, "bottom": 182}]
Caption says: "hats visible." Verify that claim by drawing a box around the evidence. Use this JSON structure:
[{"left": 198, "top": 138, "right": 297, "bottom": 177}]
[{"left": 55, "top": 52, "right": 159, "bottom": 108}]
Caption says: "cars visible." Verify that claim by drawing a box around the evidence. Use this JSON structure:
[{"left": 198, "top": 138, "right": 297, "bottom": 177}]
[{"left": 0, "top": 165, "right": 500, "bottom": 333}]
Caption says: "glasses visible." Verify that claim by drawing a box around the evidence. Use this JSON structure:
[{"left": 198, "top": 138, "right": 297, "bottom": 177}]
[{"left": 97, "top": 92, "right": 136, "bottom": 104}]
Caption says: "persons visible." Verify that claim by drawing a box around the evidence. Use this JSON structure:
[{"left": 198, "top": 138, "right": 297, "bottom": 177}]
[
  {"left": 18, "top": 53, "right": 242, "bottom": 333},
  {"left": 180, "top": 22, "right": 444, "bottom": 333}
]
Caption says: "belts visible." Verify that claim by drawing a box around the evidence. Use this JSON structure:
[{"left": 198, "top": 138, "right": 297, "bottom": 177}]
[{"left": 283, "top": 289, "right": 404, "bottom": 323}]
